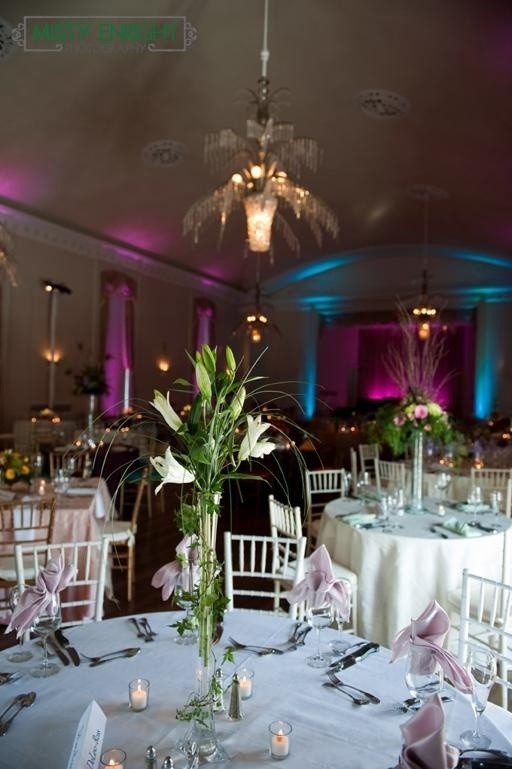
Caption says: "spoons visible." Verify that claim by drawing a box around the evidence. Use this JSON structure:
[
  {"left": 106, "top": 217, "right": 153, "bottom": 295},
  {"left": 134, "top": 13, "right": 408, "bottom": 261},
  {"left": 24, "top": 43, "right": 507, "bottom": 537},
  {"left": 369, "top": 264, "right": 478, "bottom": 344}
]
[
  {"left": 322, "top": 680, "right": 370, "bottom": 706},
  {"left": 1, "top": 690, "right": 36, "bottom": 737}
]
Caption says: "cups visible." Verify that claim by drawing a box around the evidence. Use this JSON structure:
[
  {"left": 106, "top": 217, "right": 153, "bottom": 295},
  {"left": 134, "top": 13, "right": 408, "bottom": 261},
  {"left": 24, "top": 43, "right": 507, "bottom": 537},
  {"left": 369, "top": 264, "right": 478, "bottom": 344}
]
[
  {"left": 266, "top": 720, "right": 293, "bottom": 759},
  {"left": 128, "top": 678, "right": 149, "bottom": 712},
  {"left": 235, "top": 665, "right": 254, "bottom": 700},
  {"left": 100, "top": 746, "right": 128, "bottom": 768}
]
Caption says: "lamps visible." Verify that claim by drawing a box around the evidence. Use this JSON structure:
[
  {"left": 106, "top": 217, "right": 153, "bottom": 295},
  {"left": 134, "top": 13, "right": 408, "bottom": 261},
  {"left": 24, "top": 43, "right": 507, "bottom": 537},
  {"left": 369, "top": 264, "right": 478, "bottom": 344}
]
[
  {"left": 232, "top": 260, "right": 281, "bottom": 345},
  {"left": 174, "top": 1, "right": 345, "bottom": 268},
  {"left": 394, "top": 197, "right": 456, "bottom": 344}
]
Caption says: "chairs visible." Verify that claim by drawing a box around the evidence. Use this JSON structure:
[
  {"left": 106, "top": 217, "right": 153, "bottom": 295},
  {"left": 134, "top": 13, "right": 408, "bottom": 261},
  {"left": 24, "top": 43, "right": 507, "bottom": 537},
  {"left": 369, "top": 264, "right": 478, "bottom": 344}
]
[
  {"left": 16, "top": 542, "right": 111, "bottom": 640},
  {"left": 305, "top": 416, "right": 512, "bottom": 560},
  {"left": 269, "top": 493, "right": 359, "bottom": 637},
  {"left": 1, "top": 414, "right": 170, "bottom": 611},
  {"left": 222, "top": 530, "right": 309, "bottom": 618},
  {"left": 454, "top": 567, "right": 512, "bottom": 713}
]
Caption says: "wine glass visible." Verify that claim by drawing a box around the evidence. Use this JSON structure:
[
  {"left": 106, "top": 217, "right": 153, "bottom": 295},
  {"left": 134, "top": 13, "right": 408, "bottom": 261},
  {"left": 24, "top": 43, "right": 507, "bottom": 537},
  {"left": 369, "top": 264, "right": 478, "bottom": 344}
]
[
  {"left": 462, "top": 648, "right": 498, "bottom": 749},
  {"left": 171, "top": 578, "right": 200, "bottom": 646},
  {"left": 405, "top": 648, "right": 444, "bottom": 702},
  {"left": 339, "top": 469, "right": 502, "bottom": 530},
  {"left": 330, "top": 597, "right": 353, "bottom": 650},
  {"left": 304, "top": 598, "right": 335, "bottom": 670},
  {"left": 25, "top": 452, "right": 78, "bottom": 508},
  {"left": 6, "top": 585, "right": 63, "bottom": 680}
]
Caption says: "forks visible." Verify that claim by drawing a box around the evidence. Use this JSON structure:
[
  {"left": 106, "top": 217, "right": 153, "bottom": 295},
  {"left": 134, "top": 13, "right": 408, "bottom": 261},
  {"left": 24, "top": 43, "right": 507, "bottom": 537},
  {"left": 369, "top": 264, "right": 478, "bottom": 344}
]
[
  {"left": 228, "top": 637, "right": 285, "bottom": 656},
  {"left": 287, "top": 622, "right": 314, "bottom": 653},
  {"left": 393, "top": 690, "right": 455, "bottom": 715},
  {"left": 128, "top": 615, "right": 158, "bottom": 643},
  {"left": 79, "top": 646, "right": 141, "bottom": 663},
  {"left": 326, "top": 668, "right": 381, "bottom": 704}
]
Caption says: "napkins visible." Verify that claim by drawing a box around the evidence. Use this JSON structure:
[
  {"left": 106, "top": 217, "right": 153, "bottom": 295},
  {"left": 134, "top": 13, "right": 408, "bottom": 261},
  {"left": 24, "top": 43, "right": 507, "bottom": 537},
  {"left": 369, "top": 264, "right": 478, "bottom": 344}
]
[
  {"left": 282, "top": 545, "right": 350, "bottom": 623},
  {"left": 390, "top": 597, "right": 474, "bottom": 695},
  {"left": 2, "top": 552, "right": 77, "bottom": 640},
  {"left": 150, "top": 533, "right": 222, "bottom": 599},
  {"left": 400, "top": 694, "right": 459, "bottom": 768}
]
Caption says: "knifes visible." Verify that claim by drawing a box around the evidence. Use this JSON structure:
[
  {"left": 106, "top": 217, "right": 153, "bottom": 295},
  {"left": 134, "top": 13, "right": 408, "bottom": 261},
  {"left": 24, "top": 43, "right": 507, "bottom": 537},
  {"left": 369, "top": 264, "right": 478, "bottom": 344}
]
[
  {"left": 56, "top": 628, "right": 81, "bottom": 667},
  {"left": 326, "top": 640, "right": 380, "bottom": 675},
  {"left": 47, "top": 634, "right": 69, "bottom": 666}
]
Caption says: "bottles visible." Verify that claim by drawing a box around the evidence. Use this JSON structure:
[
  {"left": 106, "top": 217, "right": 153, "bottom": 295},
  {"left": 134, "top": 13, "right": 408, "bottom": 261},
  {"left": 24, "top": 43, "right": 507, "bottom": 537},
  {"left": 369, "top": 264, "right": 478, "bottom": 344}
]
[
  {"left": 211, "top": 669, "right": 226, "bottom": 713},
  {"left": 162, "top": 755, "right": 176, "bottom": 769},
  {"left": 227, "top": 674, "right": 246, "bottom": 721},
  {"left": 144, "top": 746, "right": 157, "bottom": 769}
]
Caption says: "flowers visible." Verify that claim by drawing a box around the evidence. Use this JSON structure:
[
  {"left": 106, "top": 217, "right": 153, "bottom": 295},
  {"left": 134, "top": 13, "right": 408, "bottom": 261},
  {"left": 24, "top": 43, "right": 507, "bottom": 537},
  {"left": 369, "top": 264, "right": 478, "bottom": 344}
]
[
  {"left": 55, "top": 345, "right": 337, "bottom": 723},
  {"left": 367, "top": 304, "right": 468, "bottom": 477},
  {"left": 63, "top": 348, "right": 118, "bottom": 414}
]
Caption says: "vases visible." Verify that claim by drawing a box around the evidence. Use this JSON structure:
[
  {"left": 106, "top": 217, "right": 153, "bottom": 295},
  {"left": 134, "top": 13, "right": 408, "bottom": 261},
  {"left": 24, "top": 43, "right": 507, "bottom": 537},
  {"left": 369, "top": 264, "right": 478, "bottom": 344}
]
[{"left": 86, "top": 393, "right": 97, "bottom": 437}]
